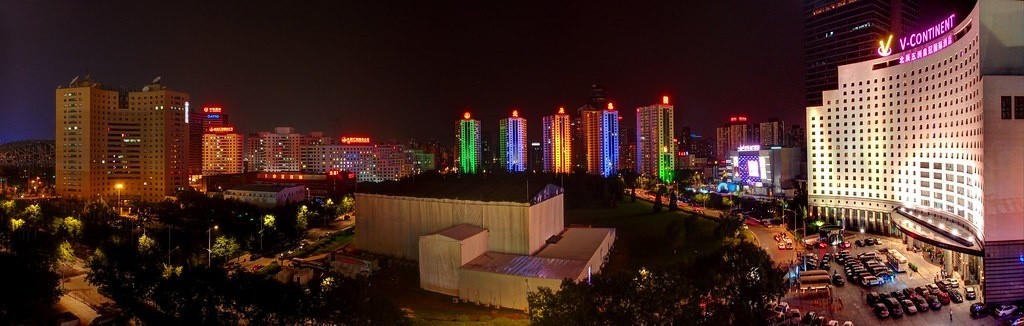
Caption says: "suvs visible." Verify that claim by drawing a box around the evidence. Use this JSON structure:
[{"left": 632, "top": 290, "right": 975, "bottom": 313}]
[{"left": 970, "top": 302, "right": 992, "bottom": 316}]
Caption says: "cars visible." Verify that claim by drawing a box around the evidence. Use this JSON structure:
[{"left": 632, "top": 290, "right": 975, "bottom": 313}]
[
  {"left": 994, "top": 304, "right": 1018, "bottom": 317},
  {"left": 804, "top": 253, "right": 834, "bottom": 270},
  {"left": 964, "top": 287, "right": 976, "bottom": 300},
  {"left": 843, "top": 320, "right": 854, "bottom": 326},
  {"left": 856, "top": 238, "right": 882, "bottom": 247},
  {"left": 774, "top": 232, "right": 793, "bottom": 250},
  {"left": 838, "top": 242, "right": 851, "bottom": 249},
  {"left": 835, "top": 250, "right": 896, "bottom": 287},
  {"left": 828, "top": 319, "right": 839, "bottom": 326},
  {"left": 868, "top": 278, "right": 963, "bottom": 319},
  {"left": 805, "top": 310, "right": 826, "bottom": 326},
  {"left": 766, "top": 300, "right": 802, "bottom": 324},
  {"left": 833, "top": 274, "right": 845, "bottom": 286}
]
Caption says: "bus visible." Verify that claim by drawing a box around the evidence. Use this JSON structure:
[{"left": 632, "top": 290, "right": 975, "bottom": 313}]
[
  {"left": 799, "top": 270, "right": 829, "bottom": 275},
  {"left": 887, "top": 249, "right": 908, "bottom": 273},
  {"left": 799, "top": 275, "right": 832, "bottom": 291}
]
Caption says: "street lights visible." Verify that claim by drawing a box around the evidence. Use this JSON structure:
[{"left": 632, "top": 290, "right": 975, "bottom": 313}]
[
  {"left": 786, "top": 208, "right": 797, "bottom": 241},
  {"left": 209, "top": 225, "right": 218, "bottom": 268}
]
